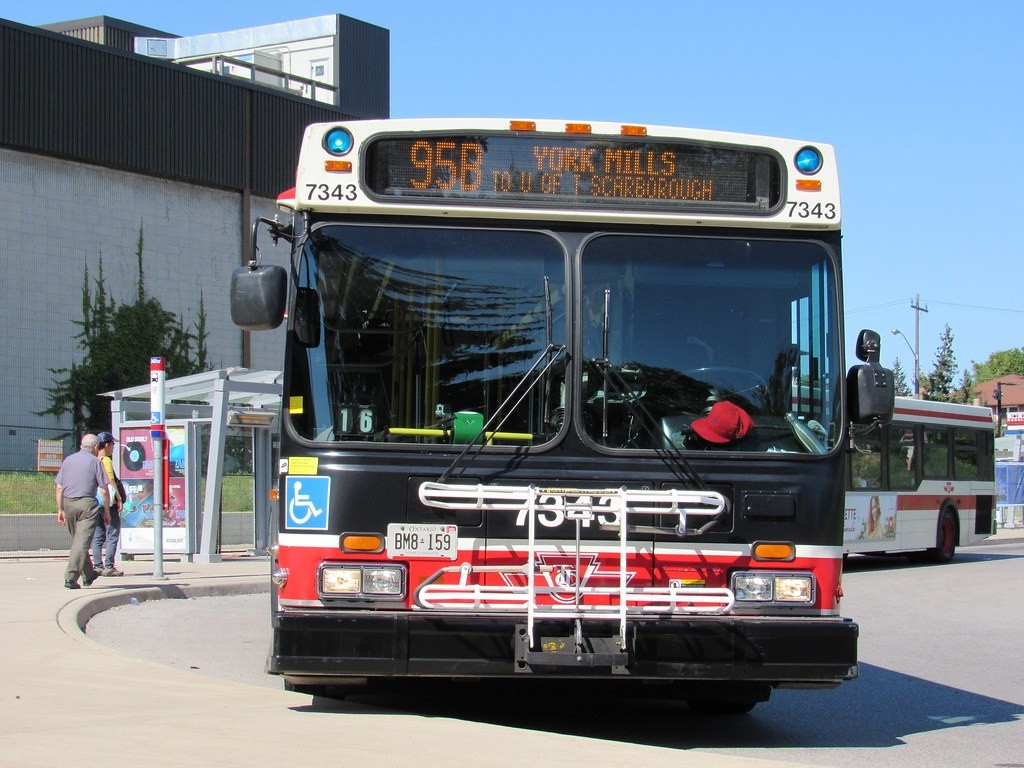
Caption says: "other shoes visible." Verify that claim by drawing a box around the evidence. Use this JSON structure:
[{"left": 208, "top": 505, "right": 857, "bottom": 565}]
[
  {"left": 83, "top": 570, "right": 99, "bottom": 586},
  {"left": 65, "top": 579, "right": 81, "bottom": 589},
  {"left": 103, "top": 567, "right": 124, "bottom": 577},
  {"left": 94, "top": 567, "right": 104, "bottom": 575}
]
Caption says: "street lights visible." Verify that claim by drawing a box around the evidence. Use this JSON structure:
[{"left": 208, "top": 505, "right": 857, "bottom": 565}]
[{"left": 891, "top": 329, "right": 919, "bottom": 400}]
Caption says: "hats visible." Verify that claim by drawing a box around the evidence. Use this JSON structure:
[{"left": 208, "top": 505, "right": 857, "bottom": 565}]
[
  {"left": 97, "top": 431, "right": 120, "bottom": 443},
  {"left": 691, "top": 400, "right": 754, "bottom": 443}
]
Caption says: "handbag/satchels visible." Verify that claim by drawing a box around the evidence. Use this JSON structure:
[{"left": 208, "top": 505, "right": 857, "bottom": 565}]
[{"left": 96, "top": 456, "right": 115, "bottom": 506}]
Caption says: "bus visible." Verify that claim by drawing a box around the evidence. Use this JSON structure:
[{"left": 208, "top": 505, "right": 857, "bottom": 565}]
[
  {"left": 231, "top": 114, "right": 897, "bottom": 716},
  {"left": 791, "top": 381, "right": 999, "bottom": 564}
]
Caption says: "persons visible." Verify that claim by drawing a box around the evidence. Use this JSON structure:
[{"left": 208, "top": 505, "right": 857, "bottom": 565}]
[
  {"left": 54, "top": 434, "right": 111, "bottom": 589},
  {"left": 92, "top": 432, "right": 124, "bottom": 576},
  {"left": 858, "top": 495, "right": 883, "bottom": 540}
]
